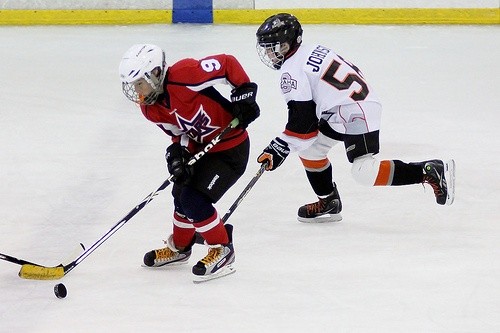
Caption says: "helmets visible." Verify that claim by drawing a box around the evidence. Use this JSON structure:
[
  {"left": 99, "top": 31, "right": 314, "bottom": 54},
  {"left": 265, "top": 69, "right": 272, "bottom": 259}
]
[
  {"left": 119, "top": 44, "right": 167, "bottom": 105},
  {"left": 255, "top": 13, "right": 303, "bottom": 71}
]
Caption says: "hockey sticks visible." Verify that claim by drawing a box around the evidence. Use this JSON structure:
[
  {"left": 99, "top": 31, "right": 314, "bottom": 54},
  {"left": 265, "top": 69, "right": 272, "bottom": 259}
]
[
  {"left": 19, "top": 117, "right": 240, "bottom": 281},
  {"left": 195, "top": 161, "right": 270, "bottom": 244}
]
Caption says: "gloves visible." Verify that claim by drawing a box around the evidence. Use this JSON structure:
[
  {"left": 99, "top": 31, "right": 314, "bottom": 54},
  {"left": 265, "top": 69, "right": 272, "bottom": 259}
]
[
  {"left": 257, "top": 137, "right": 290, "bottom": 171},
  {"left": 166, "top": 142, "right": 191, "bottom": 184},
  {"left": 231, "top": 83, "right": 260, "bottom": 129}
]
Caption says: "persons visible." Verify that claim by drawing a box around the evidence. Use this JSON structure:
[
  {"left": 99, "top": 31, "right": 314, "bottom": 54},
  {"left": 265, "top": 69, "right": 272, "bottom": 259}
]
[
  {"left": 256, "top": 13, "right": 456, "bottom": 223},
  {"left": 119, "top": 43, "right": 260, "bottom": 284}
]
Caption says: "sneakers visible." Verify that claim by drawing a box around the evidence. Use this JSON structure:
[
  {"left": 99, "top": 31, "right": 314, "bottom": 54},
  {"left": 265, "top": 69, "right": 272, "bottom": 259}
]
[
  {"left": 420, "top": 159, "right": 455, "bottom": 206},
  {"left": 192, "top": 223, "right": 235, "bottom": 282},
  {"left": 297, "top": 182, "right": 343, "bottom": 222},
  {"left": 141, "top": 231, "right": 198, "bottom": 268}
]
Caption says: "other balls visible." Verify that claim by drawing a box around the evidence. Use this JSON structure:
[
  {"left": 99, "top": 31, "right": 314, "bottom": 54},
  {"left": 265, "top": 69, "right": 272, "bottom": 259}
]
[{"left": 54, "top": 283, "right": 67, "bottom": 299}]
